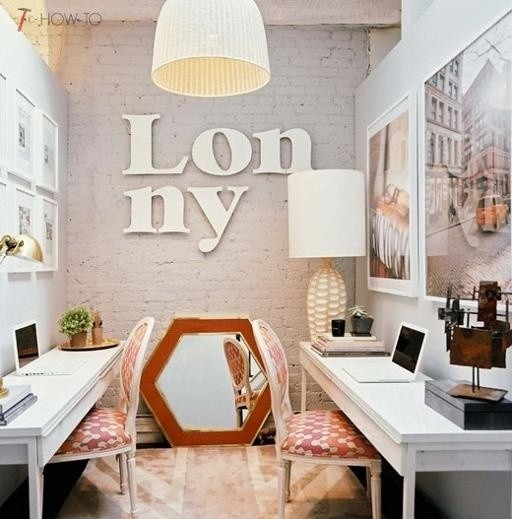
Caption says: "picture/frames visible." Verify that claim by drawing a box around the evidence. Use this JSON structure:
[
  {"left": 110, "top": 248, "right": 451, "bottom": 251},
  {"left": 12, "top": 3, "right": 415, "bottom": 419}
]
[
  {"left": 422, "top": 4, "right": 511, "bottom": 313},
  {"left": 8, "top": 185, "right": 35, "bottom": 275},
  {"left": 0, "top": 70, "right": 9, "bottom": 188},
  {"left": 36, "top": 194, "right": 59, "bottom": 272},
  {"left": 35, "top": 112, "right": 60, "bottom": 194},
  {"left": 366, "top": 89, "right": 421, "bottom": 298},
  {"left": 7, "top": 87, "right": 36, "bottom": 183},
  {"left": 0, "top": 178, "right": 11, "bottom": 276}
]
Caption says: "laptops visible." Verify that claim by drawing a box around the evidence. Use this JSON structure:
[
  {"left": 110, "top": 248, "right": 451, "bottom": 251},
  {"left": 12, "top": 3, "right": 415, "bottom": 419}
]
[
  {"left": 11, "top": 321, "right": 85, "bottom": 375},
  {"left": 343, "top": 320, "right": 429, "bottom": 383}
]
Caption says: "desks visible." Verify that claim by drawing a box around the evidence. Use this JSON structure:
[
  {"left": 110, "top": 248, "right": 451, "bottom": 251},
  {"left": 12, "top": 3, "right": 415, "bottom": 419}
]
[
  {"left": 248, "top": 369, "right": 267, "bottom": 391},
  {"left": 0, "top": 340, "right": 125, "bottom": 519},
  {"left": 298, "top": 340, "right": 512, "bottom": 519}
]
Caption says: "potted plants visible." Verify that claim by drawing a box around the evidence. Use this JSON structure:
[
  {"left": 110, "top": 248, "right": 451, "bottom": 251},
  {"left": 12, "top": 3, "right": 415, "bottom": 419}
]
[
  {"left": 345, "top": 300, "right": 377, "bottom": 337},
  {"left": 90, "top": 307, "right": 105, "bottom": 345},
  {"left": 55, "top": 306, "right": 93, "bottom": 346}
]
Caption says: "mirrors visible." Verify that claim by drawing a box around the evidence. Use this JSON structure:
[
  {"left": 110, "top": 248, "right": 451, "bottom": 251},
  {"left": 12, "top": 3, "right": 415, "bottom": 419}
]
[{"left": 140, "top": 312, "right": 273, "bottom": 447}]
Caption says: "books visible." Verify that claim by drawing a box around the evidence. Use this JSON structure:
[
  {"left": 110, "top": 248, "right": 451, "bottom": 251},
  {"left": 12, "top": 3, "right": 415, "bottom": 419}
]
[
  {"left": 0, "top": 384, "right": 39, "bottom": 429},
  {"left": 310, "top": 332, "right": 390, "bottom": 360}
]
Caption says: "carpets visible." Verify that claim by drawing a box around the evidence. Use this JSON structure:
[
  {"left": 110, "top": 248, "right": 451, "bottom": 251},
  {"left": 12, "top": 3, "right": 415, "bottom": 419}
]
[{"left": 57, "top": 445, "right": 374, "bottom": 519}]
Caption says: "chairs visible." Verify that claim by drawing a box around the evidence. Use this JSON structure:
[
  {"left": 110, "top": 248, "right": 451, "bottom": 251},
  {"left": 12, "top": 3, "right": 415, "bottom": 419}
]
[
  {"left": 222, "top": 338, "right": 258, "bottom": 429},
  {"left": 253, "top": 318, "right": 385, "bottom": 518},
  {"left": 45, "top": 316, "right": 158, "bottom": 519}
]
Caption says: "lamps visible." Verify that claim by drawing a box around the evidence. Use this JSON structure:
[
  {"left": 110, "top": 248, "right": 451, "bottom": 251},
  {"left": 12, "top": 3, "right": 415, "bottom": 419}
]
[
  {"left": 0, "top": 234, "right": 43, "bottom": 272},
  {"left": 150, "top": 0, "right": 271, "bottom": 98},
  {"left": 287, "top": 169, "right": 368, "bottom": 339}
]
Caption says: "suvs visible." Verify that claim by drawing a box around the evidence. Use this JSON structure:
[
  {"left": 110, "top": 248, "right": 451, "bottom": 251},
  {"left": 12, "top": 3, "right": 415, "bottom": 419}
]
[{"left": 474, "top": 193, "right": 510, "bottom": 234}]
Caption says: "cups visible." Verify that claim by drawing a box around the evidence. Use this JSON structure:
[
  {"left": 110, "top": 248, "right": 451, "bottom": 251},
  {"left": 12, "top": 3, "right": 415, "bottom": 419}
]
[{"left": 331, "top": 319, "right": 346, "bottom": 337}]
